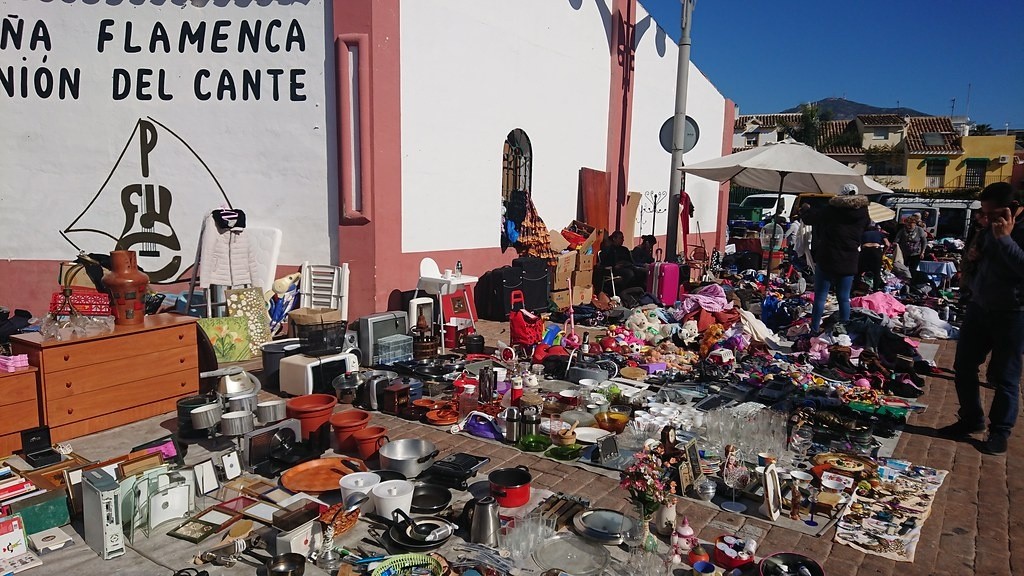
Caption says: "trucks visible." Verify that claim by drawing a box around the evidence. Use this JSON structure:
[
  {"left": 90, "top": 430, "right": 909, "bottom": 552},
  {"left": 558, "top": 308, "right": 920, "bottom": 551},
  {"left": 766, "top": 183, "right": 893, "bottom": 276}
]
[{"left": 877, "top": 194, "right": 981, "bottom": 241}]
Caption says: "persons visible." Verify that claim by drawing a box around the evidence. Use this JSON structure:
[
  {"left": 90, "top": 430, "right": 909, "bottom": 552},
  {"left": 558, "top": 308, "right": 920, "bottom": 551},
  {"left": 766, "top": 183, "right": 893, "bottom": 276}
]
[
  {"left": 800, "top": 183, "right": 871, "bottom": 339},
  {"left": 604, "top": 230, "right": 656, "bottom": 287},
  {"left": 853, "top": 230, "right": 885, "bottom": 292},
  {"left": 937, "top": 182, "right": 1024, "bottom": 456},
  {"left": 785, "top": 214, "right": 801, "bottom": 259},
  {"left": 901, "top": 216, "right": 927, "bottom": 273}
]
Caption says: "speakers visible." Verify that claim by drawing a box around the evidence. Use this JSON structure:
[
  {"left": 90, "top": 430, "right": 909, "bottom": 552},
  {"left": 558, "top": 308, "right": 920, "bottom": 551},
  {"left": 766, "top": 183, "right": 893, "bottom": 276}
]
[{"left": 341, "top": 329, "right": 363, "bottom": 367}]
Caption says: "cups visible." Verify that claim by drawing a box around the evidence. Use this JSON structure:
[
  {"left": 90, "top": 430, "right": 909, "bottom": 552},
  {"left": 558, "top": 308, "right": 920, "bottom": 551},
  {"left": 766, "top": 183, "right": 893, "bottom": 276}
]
[
  {"left": 587, "top": 404, "right": 600, "bottom": 415},
  {"left": 789, "top": 470, "right": 814, "bottom": 489},
  {"left": 759, "top": 453, "right": 777, "bottom": 467},
  {"left": 445, "top": 270, "right": 453, "bottom": 278},
  {"left": 693, "top": 561, "right": 716, "bottom": 576},
  {"left": 595, "top": 400, "right": 610, "bottom": 413}
]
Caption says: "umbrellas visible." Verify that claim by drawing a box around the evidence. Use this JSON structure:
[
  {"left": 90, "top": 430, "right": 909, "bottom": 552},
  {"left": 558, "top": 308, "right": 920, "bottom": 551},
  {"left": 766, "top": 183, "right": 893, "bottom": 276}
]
[{"left": 678, "top": 136, "right": 893, "bottom": 290}]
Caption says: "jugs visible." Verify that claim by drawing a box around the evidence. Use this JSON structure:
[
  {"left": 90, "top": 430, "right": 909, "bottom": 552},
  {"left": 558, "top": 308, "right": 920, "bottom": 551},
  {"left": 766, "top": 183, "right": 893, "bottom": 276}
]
[
  {"left": 468, "top": 495, "right": 501, "bottom": 547},
  {"left": 458, "top": 384, "right": 478, "bottom": 424}
]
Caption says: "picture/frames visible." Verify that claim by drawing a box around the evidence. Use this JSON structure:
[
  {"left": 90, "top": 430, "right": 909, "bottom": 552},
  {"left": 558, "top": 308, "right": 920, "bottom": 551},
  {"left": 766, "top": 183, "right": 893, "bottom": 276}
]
[
  {"left": 27, "top": 527, "right": 75, "bottom": 557},
  {"left": 679, "top": 438, "right": 704, "bottom": 497},
  {"left": 442, "top": 285, "right": 478, "bottom": 323},
  {"left": 759, "top": 464, "right": 782, "bottom": 521},
  {"left": 2, "top": 435, "right": 188, "bottom": 516},
  {"left": 166, "top": 476, "right": 295, "bottom": 544},
  {"left": 596, "top": 431, "right": 620, "bottom": 465}
]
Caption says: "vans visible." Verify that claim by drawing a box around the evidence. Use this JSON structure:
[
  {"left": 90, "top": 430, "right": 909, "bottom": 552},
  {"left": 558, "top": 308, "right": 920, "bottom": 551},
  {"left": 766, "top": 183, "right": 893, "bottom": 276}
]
[{"left": 740, "top": 193, "right": 797, "bottom": 223}]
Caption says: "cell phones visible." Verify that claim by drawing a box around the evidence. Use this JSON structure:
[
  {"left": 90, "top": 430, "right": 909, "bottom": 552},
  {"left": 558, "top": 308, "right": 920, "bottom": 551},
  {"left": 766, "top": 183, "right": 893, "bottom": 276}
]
[{"left": 1003, "top": 201, "right": 1020, "bottom": 220}]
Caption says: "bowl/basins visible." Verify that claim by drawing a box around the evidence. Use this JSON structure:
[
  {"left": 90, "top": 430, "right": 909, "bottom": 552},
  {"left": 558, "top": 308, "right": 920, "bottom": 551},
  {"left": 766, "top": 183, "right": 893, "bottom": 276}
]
[{"left": 579, "top": 378, "right": 598, "bottom": 392}]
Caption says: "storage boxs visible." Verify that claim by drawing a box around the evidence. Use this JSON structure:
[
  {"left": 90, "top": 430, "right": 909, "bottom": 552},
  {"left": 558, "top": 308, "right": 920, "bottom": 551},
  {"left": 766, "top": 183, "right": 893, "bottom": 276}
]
[
  {"left": 50, "top": 292, "right": 111, "bottom": 316},
  {"left": 273, "top": 498, "right": 323, "bottom": 558},
  {"left": 549, "top": 220, "right": 596, "bottom": 308},
  {"left": 706, "top": 348, "right": 735, "bottom": 371},
  {"left": 437, "top": 324, "right": 474, "bottom": 348},
  {"left": 288, "top": 307, "right": 340, "bottom": 332}
]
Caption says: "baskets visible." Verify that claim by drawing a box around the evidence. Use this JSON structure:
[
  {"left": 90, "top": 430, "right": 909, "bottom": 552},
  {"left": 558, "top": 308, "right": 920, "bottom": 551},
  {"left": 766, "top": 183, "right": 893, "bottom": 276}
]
[{"left": 48, "top": 291, "right": 112, "bottom": 316}]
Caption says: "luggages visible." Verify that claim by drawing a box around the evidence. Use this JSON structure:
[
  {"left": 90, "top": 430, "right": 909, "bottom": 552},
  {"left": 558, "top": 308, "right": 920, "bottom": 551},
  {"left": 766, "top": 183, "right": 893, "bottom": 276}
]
[
  {"left": 500, "top": 290, "right": 542, "bottom": 361},
  {"left": 512, "top": 253, "right": 552, "bottom": 314},
  {"left": 491, "top": 263, "right": 525, "bottom": 321}
]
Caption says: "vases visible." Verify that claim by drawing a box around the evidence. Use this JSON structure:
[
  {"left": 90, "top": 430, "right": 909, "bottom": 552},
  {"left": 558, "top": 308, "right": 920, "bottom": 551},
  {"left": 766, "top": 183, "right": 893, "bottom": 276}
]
[
  {"left": 638, "top": 498, "right": 677, "bottom": 553},
  {"left": 287, "top": 394, "right": 387, "bottom": 461}
]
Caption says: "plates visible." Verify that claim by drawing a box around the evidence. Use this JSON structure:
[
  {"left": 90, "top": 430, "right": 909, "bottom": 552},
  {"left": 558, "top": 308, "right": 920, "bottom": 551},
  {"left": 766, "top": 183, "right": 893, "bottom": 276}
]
[{"left": 573, "top": 427, "right": 611, "bottom": 444}]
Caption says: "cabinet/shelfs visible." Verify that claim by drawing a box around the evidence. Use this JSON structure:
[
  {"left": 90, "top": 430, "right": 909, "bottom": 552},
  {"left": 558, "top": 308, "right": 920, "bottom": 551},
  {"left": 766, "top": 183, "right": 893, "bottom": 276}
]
[
  {"left": 0, "top": 312, "right": 199, "bottom": 457},
  {"left": 382, "top": 384, "right": 410, "bottom": 416}
]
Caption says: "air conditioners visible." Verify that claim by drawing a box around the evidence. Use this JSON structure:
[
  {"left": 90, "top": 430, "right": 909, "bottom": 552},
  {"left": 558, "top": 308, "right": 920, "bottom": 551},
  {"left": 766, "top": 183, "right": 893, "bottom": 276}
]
[{"left": 999, "top": 155, "right": 1009, "bottom": 163}]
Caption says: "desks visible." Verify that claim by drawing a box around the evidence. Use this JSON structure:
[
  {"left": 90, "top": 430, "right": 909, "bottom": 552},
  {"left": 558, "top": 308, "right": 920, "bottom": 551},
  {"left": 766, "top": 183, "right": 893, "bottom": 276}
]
[{"left": 916, "top": 261, "right": 958, "bottom": 289}]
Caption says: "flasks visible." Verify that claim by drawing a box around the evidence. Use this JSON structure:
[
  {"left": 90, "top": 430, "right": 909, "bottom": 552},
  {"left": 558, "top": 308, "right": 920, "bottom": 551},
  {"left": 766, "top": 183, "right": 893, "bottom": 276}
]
[
  {"left": 519, "top": 404, "right": 539, "bottom": 440},
  {"left": 504, "top": 405, "right": 521, "bottom": 442}
]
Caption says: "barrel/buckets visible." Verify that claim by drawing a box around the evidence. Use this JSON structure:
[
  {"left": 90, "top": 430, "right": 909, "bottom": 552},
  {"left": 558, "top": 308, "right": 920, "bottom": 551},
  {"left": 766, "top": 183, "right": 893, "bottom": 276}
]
[
  {"left": 338, "top": 471, "right": 380, "bottom": 516},
  {"left": 352, "top": 426, "right": 388, "bottom": 462},
  {"left": 373, "top": 479, "right": 415, "bottom": 523},
  {"left": 329, "top": 410, "right": 371, "bottom": 454},
  {"left": 287, "top": 394, "right": 336, "bottom": 441}
]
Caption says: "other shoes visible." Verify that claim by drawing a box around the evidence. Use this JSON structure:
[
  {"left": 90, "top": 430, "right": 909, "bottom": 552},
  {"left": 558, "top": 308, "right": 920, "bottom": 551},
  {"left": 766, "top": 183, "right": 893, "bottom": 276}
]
[
  {"left": 940, "top": 416, "right": 986, "bottom": 436},
  {"left": 799, "top": 345, "right": 937, "bottom": 398},
  {"left": 985, "top": 428, "right": 1010, "bottom": 455}
]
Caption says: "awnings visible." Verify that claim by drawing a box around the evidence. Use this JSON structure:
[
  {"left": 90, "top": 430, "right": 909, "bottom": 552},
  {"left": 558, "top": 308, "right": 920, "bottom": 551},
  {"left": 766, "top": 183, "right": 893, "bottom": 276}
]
[
  {"left": 966, "top": 157, "right": 989, "bottom": 161},
  {"left": 925, "top": 157, "right": 949, "bottom": 160}
]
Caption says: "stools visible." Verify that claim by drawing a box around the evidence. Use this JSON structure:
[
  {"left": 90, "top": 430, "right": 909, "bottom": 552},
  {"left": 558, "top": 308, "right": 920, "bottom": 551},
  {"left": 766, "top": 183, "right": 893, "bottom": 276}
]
[{"left": 414, "top": 257, "right": 478, "bottom": 355}]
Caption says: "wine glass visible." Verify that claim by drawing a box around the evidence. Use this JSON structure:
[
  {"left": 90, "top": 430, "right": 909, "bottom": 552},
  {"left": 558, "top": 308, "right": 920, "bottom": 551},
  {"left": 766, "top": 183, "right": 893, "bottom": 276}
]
[{"left": 787, "top": 425, "right": 813, "bottom": 470}]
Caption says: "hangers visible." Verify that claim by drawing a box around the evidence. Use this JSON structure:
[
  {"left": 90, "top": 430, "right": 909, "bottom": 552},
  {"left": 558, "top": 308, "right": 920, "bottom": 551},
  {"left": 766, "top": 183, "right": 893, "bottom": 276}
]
[{"left": 220, "top": 203, "right": 238, "bottom": 219}]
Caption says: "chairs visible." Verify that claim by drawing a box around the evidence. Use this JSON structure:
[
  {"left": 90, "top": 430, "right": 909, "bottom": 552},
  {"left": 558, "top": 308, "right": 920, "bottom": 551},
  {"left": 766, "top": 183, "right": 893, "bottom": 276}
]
[
  {"left": 597, "top": 250, "right": 622, "bottom": 296},
  {"left": 298, "top": 261, "right": 350, "bottom": 321}
]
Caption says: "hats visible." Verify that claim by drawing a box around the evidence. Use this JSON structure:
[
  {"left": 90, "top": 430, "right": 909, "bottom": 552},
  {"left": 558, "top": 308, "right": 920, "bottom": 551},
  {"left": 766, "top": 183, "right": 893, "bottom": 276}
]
[
  {"left": 904, "top": 216, "right": 918, "bottom": 223},
  {"left": 841, "top": 183, "right": 859, "bottom": 196}
]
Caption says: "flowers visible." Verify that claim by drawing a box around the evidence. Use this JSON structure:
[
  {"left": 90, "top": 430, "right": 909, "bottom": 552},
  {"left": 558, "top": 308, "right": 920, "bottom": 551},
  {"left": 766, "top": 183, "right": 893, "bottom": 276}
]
[{"left": 618, "top": 450, "right": 678, "bottom": 522}]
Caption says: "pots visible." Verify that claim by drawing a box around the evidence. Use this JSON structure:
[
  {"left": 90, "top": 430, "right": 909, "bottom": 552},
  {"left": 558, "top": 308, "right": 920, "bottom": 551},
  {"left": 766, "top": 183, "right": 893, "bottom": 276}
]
[{"left": 174, "top": 338, "right": 642, "bottom": 575}]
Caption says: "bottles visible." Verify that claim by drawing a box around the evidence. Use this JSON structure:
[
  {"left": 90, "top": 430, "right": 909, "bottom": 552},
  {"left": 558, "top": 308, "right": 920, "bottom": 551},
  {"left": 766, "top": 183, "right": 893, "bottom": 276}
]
[
  {"left": 101, "top": 250, "right": 149, "bottom": 326},
  {"left": 580, "top": 332, "right": 591, "bottom": 354},
  {"left": 456, "top": 261, "right": 462, "bottom": 278}
]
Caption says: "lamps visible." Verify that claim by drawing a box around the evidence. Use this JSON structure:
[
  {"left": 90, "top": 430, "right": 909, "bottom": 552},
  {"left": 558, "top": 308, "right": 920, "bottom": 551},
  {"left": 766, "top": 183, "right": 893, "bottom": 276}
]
[
  {"left": 316, "top": 493, "right": 370, "bottom": 568},
  {"left": 58, "top": 250, "right": 100, "bottom": 318},
  {"left": 191, "top": 393, "right": 287, "bottom": 453}
]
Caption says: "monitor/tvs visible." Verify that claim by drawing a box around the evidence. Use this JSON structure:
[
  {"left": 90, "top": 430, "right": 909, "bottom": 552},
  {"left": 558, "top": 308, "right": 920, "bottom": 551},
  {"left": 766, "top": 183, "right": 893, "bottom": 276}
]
[{"left": 354, "top": 310, "right": 408, "bottom": 367}]
